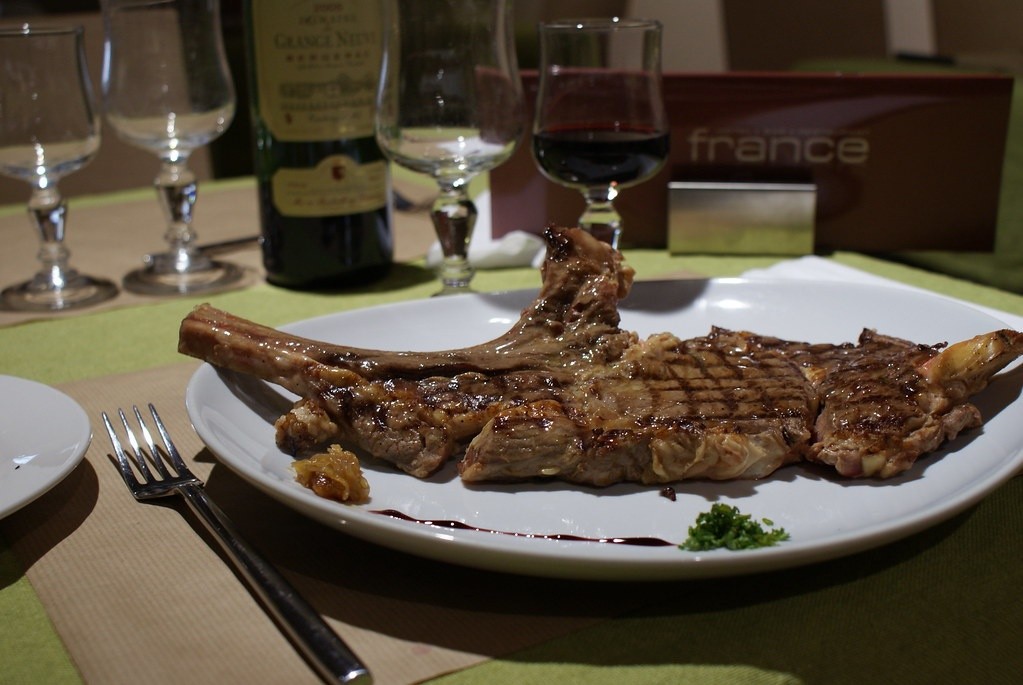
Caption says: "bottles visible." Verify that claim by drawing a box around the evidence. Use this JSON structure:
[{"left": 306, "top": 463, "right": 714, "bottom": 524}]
[{"left": 253, "top": 0, "right": 400, "bottom": 296}]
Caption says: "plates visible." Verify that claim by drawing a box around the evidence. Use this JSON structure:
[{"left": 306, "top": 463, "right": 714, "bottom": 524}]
[
  {"left": 0, "top": 373, "right": 92, "bottom": 519},
  {"left": 184, "top": 276, "right": 1023, "bottom": 582}
]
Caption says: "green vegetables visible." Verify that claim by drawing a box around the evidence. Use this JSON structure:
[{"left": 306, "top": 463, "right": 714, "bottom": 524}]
[{"left": 676, "top": 501, "right": 790, "bottom": 552}]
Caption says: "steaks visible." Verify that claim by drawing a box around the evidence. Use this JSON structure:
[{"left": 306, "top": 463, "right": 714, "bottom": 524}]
[{"left": 174, "top": 222, "right": 1023, "bottom": 479}]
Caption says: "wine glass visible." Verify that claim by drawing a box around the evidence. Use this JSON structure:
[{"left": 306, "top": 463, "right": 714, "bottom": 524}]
[
  {"left": 530, "top": 18, "right": 673, "bottom": 253},
  {"left": 98, "top": 0, "right": 259, "bottom": 298},
  {"left": 374, "top": 0, "right": 530, "bottom": 297},
  {"left": 0, "top": 25, "right": 120, "bottom": 313}
]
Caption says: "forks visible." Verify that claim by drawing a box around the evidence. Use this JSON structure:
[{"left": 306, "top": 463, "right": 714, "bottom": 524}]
[{"left": 102, "top": 402, "right": 372, "bottom": 685}]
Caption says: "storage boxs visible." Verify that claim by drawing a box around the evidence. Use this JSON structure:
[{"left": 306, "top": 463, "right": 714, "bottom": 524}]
[{"left": 475, "top": 50, "right": 1016, "bottom": 255}]
